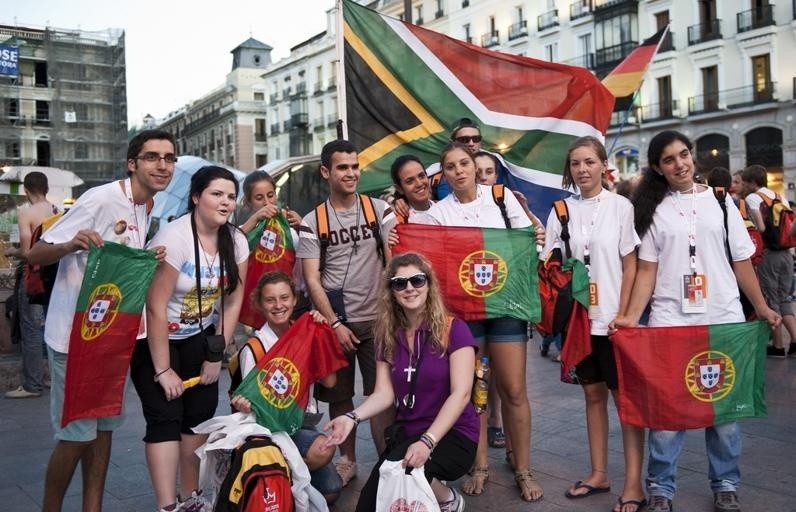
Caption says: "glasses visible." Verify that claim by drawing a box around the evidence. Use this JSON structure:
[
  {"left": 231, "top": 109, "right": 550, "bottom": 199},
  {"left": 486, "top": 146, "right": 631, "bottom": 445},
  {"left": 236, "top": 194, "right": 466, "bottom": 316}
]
[
  {"left": 452, "top": 135, "right": 481, "bottom": 144},
  {"left": 130, "top": 153, "right": 177, "bottom": 164},
  {"left": 389, "top": 273, "right": 428, "bottom": 292}
]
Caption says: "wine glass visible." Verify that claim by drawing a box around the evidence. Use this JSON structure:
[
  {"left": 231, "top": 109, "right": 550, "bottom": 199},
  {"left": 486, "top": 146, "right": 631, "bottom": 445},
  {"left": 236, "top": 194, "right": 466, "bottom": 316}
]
[{"left": 6, "top": 256, "right": 14, "bottom": 270}]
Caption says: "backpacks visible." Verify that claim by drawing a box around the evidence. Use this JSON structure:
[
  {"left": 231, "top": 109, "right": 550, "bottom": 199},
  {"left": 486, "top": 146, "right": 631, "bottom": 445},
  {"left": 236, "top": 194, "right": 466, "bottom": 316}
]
[
  {"left": 23, "top": 209, "right": 73, "bottom": 306},
  {"left": 754, "top": 189, "right": 796, "bottom": 253},
  {"left": 738, "top": 198, "right": 765, "bottom": 266},
  {"left": 530, "top": 198, "right": 578, "bottom": 339},
  {"left": 210, "top": 430, "right": 295, "bottom": 512}
]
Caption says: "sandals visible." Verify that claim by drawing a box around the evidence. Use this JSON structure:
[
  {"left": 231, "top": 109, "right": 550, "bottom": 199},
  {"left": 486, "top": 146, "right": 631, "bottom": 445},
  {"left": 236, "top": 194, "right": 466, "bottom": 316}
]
[
  {"left": 514, "top": 469, "right": 544, "bottom": 503},
  {"left": 460, "top": 464, "right": 491, "bottom": 497},
  {"left": 505, "top": 451, "right": 519, "bottom": 473}
]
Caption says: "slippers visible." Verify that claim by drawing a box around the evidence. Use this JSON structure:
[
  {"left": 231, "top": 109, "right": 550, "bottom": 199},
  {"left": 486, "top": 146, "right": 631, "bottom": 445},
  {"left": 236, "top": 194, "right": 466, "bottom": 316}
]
[
  {"left": 564, "top": 480, "right": 612, "bottom": 499},
  {"left": 612, "top": 495, "right": 648, "bottom": 512}
]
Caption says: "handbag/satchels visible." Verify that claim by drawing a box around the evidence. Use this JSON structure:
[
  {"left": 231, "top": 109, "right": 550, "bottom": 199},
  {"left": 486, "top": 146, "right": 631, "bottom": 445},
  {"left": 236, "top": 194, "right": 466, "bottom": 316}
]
[
  {"left": 381, "top": 422, "right": 409, "bottom": 462},
  {"left": 205, "top": 335, "right": 226, "bottom": 364},
  {"left": 375, "top": 457, "right": 441, "bottom": 512},
  {"left": 325, "top": 287, "right": 347, "bottom": 324}
]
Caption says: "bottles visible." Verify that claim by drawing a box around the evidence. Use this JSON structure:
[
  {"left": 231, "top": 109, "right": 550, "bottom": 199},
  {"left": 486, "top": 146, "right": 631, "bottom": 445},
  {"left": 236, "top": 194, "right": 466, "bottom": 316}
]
[{"left": 474, "top": 356, "right": 492, "bottom": 413}]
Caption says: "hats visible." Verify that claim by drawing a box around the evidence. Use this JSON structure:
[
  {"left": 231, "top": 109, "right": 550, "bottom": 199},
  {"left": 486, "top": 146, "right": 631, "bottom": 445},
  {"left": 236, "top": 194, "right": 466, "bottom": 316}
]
[{"left": 451, "top": 117, "right": 481, "bottom": 135}]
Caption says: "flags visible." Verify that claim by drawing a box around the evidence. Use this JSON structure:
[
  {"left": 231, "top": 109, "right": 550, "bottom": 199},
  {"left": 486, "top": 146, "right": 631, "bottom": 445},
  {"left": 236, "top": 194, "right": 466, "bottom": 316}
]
[
  {"left": 340, "top": 1, "right": 617, "bottom": 225},
  {"left": 601, "top": 23, "right": 669, "bottom": 110}
]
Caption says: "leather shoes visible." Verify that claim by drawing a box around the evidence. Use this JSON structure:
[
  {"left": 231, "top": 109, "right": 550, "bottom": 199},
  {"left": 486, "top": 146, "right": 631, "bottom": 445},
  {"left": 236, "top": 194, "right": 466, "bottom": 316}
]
[{"left": 4, "top": 385, "right": 42, "bottom": 399}]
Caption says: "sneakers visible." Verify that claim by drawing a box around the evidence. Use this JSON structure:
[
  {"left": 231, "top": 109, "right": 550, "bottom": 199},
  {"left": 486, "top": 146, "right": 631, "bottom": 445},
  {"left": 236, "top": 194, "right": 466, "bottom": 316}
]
[
  {"left": 439, "top": 486, "right": 466, "bottom": 512},
  {"left": 175, "top": 489, "right": 213, "bottom": 512},
  {"left": 712, "top": 490, "right": 742, "bottom": 512},
  {"left": 647, "top": 493, "right": 673, "bottom": 512},
  {"left": 767, "top": 344, "right": 786, "bottom": 359},
  {"left": 333, "top": 453, "right": 358, "bottom": 488},
  {"left": 159, "top": 497, "right": 192, "bottom": 512},
  {"left": 489, "top": 428, "right": 505, "bottom": 448},
  {"left": 787, "top": 341, "right": 796, "bottom": 358},
  {"left": 538, "top": 343, "right": 549, "bottom": 358}
]
